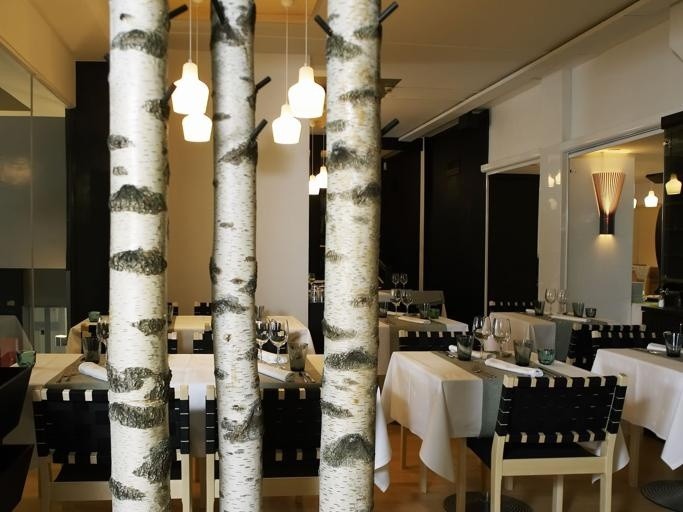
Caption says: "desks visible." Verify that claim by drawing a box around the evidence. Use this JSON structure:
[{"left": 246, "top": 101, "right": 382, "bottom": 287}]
[{"left": 0, "top": 350, "right": 323, "bottom": 507}]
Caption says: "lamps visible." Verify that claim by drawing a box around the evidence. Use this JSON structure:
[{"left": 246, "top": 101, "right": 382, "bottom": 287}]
[
  {"left": 591, "top": 169, "right": 626, "bottom": 236},
  {"left": 665, "top": 171, "right": 682, "bottom": 195},
  {"left": 642, "top": 189, "right": 659, "bottom": 209},
  {"left": 270, "top": 0, "right": 326, "bottom": 146},
  {"left": 168, "top": 1, "right": 213, "bottom": 146}
]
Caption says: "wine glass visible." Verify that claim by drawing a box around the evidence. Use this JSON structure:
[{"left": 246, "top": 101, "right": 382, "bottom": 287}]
[
  {"left": 254, "top": 319, "right": 272, "bottom": 364},
  {"left": 471, "top": 315, "right": 492, "bottom": 360},
  {"left": 492, "top": 317, "right": 513, "bottom": 359},
  {"left": 544, "top": 287, "right": 559, "bottom": 316},
  {"left": 556, "top": 288, "right": 570, "bottom": 315},
  {"left": 267, "top": 318, "right": 290, "bottom": 364},
  {"left": 390, "top": 272, "right": 417, "bottom": 316}
]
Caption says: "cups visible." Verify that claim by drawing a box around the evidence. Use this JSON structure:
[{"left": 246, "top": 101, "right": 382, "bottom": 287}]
[
  {"left": 584, "top": 307, "right": 598, "bottom": 319},
  {"left": 536, "top": 347, "right": 557, "bottom": 366},
  {"left": 287, "top": 341, "right": 311, "bottom": 373},
  {"left": 663, "top": 330, "right": 683, "bottom": 358},
  {"left": 572, "top": 302, "right": 584, "bottom": 318},
  {"left": 418, "top": 302, "right": 432, "bottom": 320},
  {"left": 513, "top": 338, "right": 534, "bottom": 368},
  {"left": 88, "top": 311, "right": 100, "bottom": 322},
  {"left": 14, "top": 350, "right": 37, "bottom": 370},
  {"left": 456, "top": 334, "right": 475, "bottom": 362}
]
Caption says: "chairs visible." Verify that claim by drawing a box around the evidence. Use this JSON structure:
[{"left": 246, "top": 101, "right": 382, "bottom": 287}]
[
  {"left": 31, "top": 299, "right": 322, "bottom": 511},
  {"left": 377, "top": 289, "right": 661, "bottom": 512}
]
